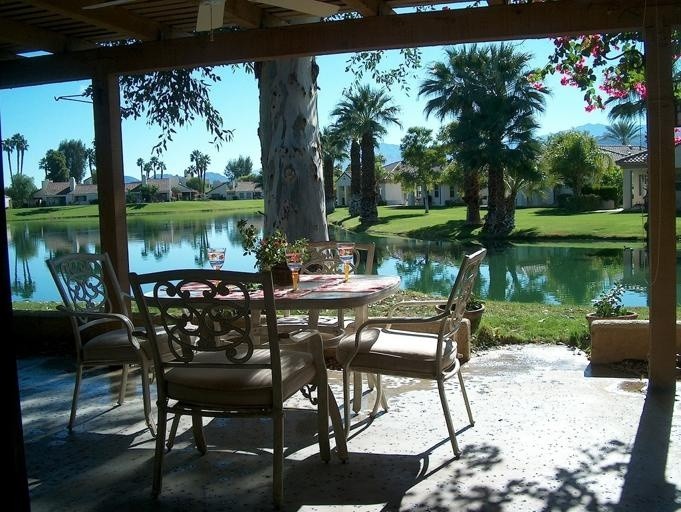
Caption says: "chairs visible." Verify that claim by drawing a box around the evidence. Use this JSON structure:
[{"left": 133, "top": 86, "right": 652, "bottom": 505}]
[
  {"left": 45, "top": 252, "right": 196, "bottom": 438},
  {"left": 143, "top": 242, "right": 401, "bottom": 444},
  {"left": 127, "top": 268, "right": 330, "bottom": 507},
  {"left": 336, "top": 248, "right": 488, "bottom": 456}
]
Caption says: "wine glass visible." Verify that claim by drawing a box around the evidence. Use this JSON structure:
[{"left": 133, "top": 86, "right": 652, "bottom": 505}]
[
  {"left": 285, "top": 247, "right": 305, "bottom": 292},
  {"left": 206, "top": 247, "right": 227, "bottom": 294},
  {"left": 337, "top": 242, "right": 356, "bottom": 283}
]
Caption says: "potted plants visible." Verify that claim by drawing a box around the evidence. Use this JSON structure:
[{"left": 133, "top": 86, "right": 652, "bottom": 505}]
[{"left": 585, "top": 281, "right": 638, "bottom": 335}]
